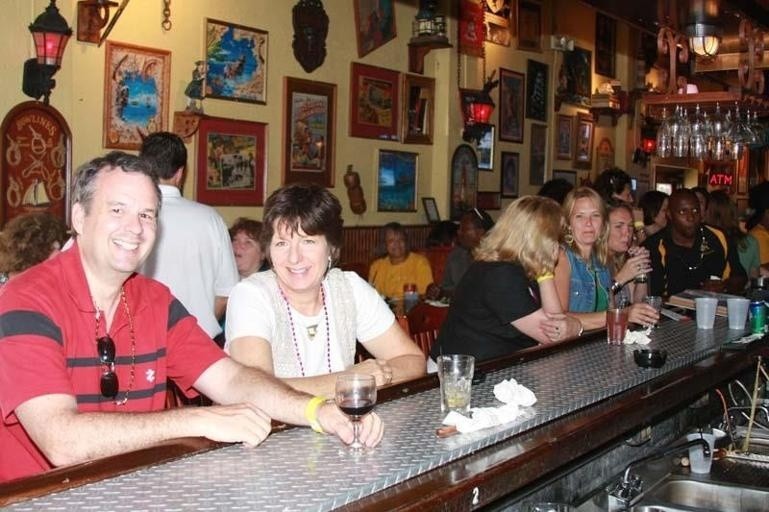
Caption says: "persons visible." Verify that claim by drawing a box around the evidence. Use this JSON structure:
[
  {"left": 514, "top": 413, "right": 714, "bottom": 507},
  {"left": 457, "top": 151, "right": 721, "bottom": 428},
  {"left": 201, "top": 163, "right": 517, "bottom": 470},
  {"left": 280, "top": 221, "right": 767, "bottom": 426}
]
[
  {"left": 367, "top": 223, "right": 434, "bottom": 299},
  {"left": 212, "top": 184, "right": 426, "bottom": 406},
  {"left": 0, "top": 212, "right": 71, "bottom": 287},
  {"left": 0, "top": 151, "right": 384, "bottom": 483},
  {"left": 61, "top": 132, "right": 240, "bottom": 351},
  {"left": 213, "top": 217, "right": 273, "bottom": 334}
]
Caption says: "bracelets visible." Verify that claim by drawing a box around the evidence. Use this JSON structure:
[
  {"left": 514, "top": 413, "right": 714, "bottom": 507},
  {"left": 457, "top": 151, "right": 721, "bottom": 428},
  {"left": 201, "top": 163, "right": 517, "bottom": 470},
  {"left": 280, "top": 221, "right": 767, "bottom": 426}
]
[{"left": 304, "top": 395, "right": 334, "bottom": 435}]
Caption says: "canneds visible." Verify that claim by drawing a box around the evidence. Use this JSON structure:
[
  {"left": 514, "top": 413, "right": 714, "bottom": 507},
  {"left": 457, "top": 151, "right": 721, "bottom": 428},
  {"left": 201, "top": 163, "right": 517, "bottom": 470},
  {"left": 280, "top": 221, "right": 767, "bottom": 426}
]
[{"left": 749, "top": 301, "right": 766, "bottom": 334}]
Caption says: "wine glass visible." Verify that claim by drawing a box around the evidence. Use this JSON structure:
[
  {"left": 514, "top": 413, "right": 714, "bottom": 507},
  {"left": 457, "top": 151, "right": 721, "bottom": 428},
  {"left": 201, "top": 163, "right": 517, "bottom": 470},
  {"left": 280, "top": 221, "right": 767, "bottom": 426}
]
[
  {"left": 655, "top": 103, "right": 766, "bottom": 160},
  {"left": 336, "top": 375, "right": 379, "bottom": 452}
]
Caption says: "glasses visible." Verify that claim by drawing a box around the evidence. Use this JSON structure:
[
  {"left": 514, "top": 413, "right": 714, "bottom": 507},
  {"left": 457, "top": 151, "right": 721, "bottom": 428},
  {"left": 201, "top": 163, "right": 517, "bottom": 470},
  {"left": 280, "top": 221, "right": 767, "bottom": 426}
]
[{"left": 96, "top": 336, "right": 120, "bottom": 401}]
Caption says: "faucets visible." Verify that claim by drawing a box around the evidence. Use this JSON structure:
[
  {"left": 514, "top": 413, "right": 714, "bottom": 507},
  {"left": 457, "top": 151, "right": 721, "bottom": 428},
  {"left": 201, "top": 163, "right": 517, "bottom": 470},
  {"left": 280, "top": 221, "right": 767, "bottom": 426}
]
[{"left": 615, "top": 405, "right": 769, "bottom": 498}]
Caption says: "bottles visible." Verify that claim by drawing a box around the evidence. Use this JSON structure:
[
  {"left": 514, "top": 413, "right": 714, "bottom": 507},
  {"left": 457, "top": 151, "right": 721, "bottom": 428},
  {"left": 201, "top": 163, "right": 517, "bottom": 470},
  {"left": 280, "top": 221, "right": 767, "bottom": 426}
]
[{"left": 404, "top": 282, "right": 419, "bottom": 309}]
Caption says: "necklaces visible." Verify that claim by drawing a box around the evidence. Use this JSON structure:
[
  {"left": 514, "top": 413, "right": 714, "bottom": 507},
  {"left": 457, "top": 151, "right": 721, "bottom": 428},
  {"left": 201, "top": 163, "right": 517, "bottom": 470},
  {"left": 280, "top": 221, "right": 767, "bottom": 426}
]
[
  {"left": 277, "top": 282, "right": 331, "bottom": 377},
  {"left": 90, "top": 287, "right": 135, "bottom": 405}
]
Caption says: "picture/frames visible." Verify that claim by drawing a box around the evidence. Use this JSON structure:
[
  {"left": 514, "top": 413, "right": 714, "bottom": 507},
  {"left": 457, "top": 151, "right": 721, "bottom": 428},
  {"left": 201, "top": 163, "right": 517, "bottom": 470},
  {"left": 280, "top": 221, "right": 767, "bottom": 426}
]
[
  {"left": 420, "top": 197, "right": 444, "bottom": 223},
  {"left": 103, "top": 1, "right": 620, "bottom": 215}
]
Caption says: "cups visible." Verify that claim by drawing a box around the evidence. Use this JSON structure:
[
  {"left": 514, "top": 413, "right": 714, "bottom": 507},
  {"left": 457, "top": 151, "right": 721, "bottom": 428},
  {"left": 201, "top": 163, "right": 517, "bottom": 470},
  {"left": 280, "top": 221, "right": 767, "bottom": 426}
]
[
  {"left": 606, "top": 292, "right": 630, "bottom": 345},
  {"left": 633, "top": 273, "right": 648, "bottom": 283},
  {"left": 687, "top": 433, "right": 715, "bottom": 473},
  {"left": 728, "top": 298, "right": 750, "bottom": 333},
  {"left": 437, "top": 355, "right": 476, "bottom": 420},
  {"left": 695, "top": 297, "right": 719, "bottom": 332},
  {"left": 643, "top": 295, "right": 663, "bottom": 329}
]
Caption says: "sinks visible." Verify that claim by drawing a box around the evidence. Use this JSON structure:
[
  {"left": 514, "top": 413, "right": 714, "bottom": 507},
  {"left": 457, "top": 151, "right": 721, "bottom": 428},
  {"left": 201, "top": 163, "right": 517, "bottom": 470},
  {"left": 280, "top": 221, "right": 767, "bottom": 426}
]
[{"left": 624, "top": 380, "right": 769, "bottom": 512}]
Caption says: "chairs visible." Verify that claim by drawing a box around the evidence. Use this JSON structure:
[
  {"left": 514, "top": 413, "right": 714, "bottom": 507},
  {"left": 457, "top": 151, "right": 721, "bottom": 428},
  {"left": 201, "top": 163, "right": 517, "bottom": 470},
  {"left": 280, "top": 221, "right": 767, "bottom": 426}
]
[{"left": 406, "top": 301, "right": 450, "bottom": 361}]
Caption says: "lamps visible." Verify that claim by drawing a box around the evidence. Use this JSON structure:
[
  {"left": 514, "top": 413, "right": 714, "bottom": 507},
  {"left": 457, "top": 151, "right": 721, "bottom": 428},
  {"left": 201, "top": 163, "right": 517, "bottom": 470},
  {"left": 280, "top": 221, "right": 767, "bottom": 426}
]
[
  {"left": 639, "top": 117, "right": 657, "bottom": 153},
  {"left": 20, "top": 0, "right": 76, "bottom": 108},
  {"left": 678, "top": 0, "right": 724, "bottom": 61}
]
